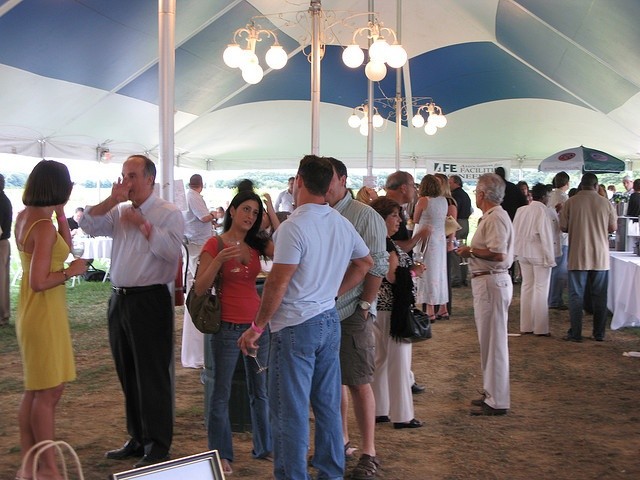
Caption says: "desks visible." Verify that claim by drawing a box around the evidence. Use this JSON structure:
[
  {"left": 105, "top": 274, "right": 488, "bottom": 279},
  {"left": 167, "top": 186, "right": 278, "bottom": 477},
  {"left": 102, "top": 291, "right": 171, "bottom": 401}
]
[
  {"left": 619, "top": 260, "right": 640, "bottom": 320},
  {"left": 606, "top": 251, "right": 640, "bottom": 331},
  {"left": 82, "top": 237, "right": 113, "bottom": 282}
]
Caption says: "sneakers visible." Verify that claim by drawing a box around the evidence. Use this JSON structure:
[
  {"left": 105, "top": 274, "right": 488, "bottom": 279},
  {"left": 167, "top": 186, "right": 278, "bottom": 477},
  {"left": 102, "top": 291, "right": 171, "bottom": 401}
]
[
  {"left": 411, "top": 384, "right": 426, "bottom": 393},
  {"left": 563, "top": 334, "right": 583, "bottom": 342},
  {"left": 549, "top": 305, "right": 568, "bottom": 310},
  {"left": 589, "top": 335, "right": 605, "bottom": 341}
]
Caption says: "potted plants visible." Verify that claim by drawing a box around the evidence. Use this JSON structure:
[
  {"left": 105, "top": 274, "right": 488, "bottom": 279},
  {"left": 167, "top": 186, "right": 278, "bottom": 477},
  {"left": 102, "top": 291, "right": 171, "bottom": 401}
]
[{"left": 613, "top": 192, "right": 629, "bottom": 216}]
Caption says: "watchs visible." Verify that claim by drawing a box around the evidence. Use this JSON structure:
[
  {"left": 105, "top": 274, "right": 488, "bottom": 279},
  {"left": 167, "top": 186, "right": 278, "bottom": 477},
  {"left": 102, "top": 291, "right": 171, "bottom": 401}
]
[
  {"left": 141, "top": 219, "right": 153, "bottom": 239},
  {"left": 358, "top": 300, "right": 371, "bottom": 312},
  {"left": 470, "top": 248, "right": 475, "bottom": 258},
  {"left": 252, "top": 321, "right": 264, "bottom": 333}
]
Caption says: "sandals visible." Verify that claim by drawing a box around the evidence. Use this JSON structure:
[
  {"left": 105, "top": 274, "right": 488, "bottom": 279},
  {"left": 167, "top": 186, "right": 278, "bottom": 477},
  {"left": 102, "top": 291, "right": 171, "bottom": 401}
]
[
  {"left": 308, "top": 441, "right": 354, "bottom": 467},
  {"left": 429, "top": 314, "right": 435, "bottom": 323},
  {"left": 350, "top": 454, "right": 380, "bottom": 480},
  {"left": 220, "top": 458, "right": 232, "bottom": 475},
  {"left": 435, "top": 312, "right": 449, "bottom": 320}
]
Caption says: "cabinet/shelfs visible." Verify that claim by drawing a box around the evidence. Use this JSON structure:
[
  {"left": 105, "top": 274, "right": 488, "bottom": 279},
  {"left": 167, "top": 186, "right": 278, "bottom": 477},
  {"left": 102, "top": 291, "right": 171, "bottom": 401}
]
[{"left": 615, "top": 216, "right": 640, "bottom": 252}]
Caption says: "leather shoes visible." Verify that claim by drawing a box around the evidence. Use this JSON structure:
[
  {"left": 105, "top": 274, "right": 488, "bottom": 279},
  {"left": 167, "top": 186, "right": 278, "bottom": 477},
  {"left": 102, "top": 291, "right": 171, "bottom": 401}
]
[
  {"left": 470, "top": 403, "right": 507, "bottom": 416},
  {"left": 525, "top": 332, "right": 533, "bottom": 334},
  {"left": 133, "top": 454, "right": 170, "bottom": 469},
  {"left": 536, "top": 333, "right": 551, "bottom": 337},
  {"left": 375, "top": 416, "right": 390, "bottom": 423},
  {"left": 472, "top": 400, "right": 485, "bottom": 406},
  {"left": 393, "top": 419, "right": 422, "bottom": 429},
  {"left": 105, "top": 438, "right": 144, "bottom": 459}
]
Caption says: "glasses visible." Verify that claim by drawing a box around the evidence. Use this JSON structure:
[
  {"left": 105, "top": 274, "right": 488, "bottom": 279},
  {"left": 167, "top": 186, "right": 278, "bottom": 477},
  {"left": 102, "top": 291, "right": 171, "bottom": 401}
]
[{"left": 473, "top": 190, "right": 480, "bottom": 194}]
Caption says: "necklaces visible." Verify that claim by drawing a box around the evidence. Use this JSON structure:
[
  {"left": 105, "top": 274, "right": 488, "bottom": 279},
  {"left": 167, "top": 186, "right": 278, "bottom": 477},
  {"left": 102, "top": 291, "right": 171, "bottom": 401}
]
[{"left": 220, "top": 237, "right": 246, "bottom": 247}]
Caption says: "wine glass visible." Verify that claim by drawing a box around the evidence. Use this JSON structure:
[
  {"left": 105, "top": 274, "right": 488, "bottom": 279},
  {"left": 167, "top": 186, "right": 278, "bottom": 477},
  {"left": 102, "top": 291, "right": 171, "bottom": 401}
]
[
  {"left": 412, "top": 252, "right": 423, "bottom": 266},
  {"left": 456, "top": 239, "right": 469, "bottom": 265},
  {"left": 72, "top": 242, "right": 84, "bottom": 260},
  {"left": 249, "top": 342, "right": 270, "bottom": 375},
  {"left": 228, "top": 239, "right": 243, "bottom": 273}
]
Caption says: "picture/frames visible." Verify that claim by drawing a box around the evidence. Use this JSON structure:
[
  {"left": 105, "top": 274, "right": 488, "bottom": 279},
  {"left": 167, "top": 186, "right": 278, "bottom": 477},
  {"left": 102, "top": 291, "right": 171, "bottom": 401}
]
[{"left": 110, "top": 449, "right": 226, "bottom": 480}]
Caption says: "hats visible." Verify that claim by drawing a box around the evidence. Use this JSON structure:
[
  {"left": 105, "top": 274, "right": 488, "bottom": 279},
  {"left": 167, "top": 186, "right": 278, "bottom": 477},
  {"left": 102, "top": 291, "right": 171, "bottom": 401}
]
[
  {"left": 598, "top": 186, "right": 606, "bottom": 198},
  {"left": 623, "top": 176, "right": 635, "bottom": 182},
  {"left": 553, "top": 172, "right": 569, "bottom": 188}
]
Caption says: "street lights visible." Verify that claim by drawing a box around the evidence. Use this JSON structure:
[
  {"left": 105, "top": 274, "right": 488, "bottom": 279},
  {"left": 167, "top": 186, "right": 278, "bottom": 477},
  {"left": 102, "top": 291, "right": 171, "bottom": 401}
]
[
  {"left": 222, "top": 0, "right": 408, "bottom": 155},
  {"left": 347, "top": 96, "right": 447, "bottom": 171}
]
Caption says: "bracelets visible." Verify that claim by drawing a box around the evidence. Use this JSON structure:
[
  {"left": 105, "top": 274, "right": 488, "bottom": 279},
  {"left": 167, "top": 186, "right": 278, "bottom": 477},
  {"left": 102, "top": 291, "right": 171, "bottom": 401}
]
[
  {"left": 61, "top": 269, "right": 69, "bottom": 280},
  {"left": 410, "top": 269, "right": 416, "bottom": 278}
]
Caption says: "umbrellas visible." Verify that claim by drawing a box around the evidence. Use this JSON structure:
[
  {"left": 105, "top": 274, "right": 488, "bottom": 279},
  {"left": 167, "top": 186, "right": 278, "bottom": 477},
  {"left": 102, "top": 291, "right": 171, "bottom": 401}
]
[{"left": 537, "top": 145, "right": 625, "bottom": 177}]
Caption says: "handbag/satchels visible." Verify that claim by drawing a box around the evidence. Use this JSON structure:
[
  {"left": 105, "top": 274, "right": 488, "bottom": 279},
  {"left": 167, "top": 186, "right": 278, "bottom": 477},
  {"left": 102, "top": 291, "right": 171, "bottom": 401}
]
[
  {"left": 445, "top": 214, "right": 462, "bottom": 237},
  {"left": 400, "top": 304, "right": 432, "bottom": 343},
  {"left": 186, "top": 236, "right": 223, "bottom": 334}
]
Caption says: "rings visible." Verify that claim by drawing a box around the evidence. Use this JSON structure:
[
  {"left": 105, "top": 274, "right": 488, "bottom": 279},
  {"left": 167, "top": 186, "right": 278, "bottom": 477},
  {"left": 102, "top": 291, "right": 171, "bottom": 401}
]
[{"left": 414, "top": 261, "right": 420, "bottom": 266}]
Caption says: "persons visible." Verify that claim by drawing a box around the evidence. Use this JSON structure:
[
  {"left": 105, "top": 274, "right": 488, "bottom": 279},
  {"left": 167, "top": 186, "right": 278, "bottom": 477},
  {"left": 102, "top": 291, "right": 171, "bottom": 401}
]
[
  {"left": 421, "top": 173, "right": 457, "bottom": 316},
  {"left": 597, "top": 184, "right": 608, "bottom": 199},
  {"left": 495, "top": 167, "right": 528, "bottom": 222},
  {"left": 0, "top": 174, "right": 14, "bottom": 324},
  {"left": 512, "top": 183, "right": 561, "bottom": 337},
  {"left": 607, "top": 185, "right": 616, "bottom": 193},
  {"left": 66, "top": 206, "right": 83, "bottom": 230},
  {"left": 14, "top": 160, "right": 94, "bottom": 479},
  {"left": 237, "top": 178, "right": 271, "bottom": 230},
  {"left": 356, "top": 186, "right": 378, "bottom": 203},
  {"left": 400, "top": 185, "right": 420, "bottom": 240},
  {"left": 622, "top": 175, "right": 634, "bottom": 193},
  {"left": 274, "top": 177, "right": 295, "bottom": 214},
  {"left": 455, "top": 173, "right": 514, "bottom": 416},
  {"left": 627, "top": 179, "right": 640, "bottom": 223},
  {"left": 180, "top": 173, "right": 217, "bottom": 295},
  {"left": 384, "top": 170, "right": 432, "bottom": 260},
  {"left": 80, "top": 154, "right": 184, "bottom": 467},
  {"left": 448, "top": 175, "right": 471, "bottom": 287},
  {"left": 193, "top": 191, "right": 281, "bottom": 475},
  {"left": 545, "top": 183, "right": 554, "bottom": 193},
  {"left": 323, "top": 158, "right": 390, "bottom": 479},
  {"left": 515, "top": 180, "right": 533, "bottom": 204},
  {"left": 544, "top": 170, "right": 569, "bottom": 310},
  {"left": 371, "top": 197, "right": 425, "bottom": 430},
  {"left": 409, "top": 174, "right": 450, "bottom": 322},
  {"left": 237, "top": 155, "right": 374, "bottom": 479},
  {"left": 559, "top": 172, "right": 617, "bottom": 342},
  {"left": 567, "top": 188, "right": 577, "bottom": 197}
]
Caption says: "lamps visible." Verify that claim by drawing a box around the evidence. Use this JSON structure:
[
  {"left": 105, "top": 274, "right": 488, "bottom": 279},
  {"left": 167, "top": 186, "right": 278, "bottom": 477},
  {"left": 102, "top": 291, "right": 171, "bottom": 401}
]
[
  {"left": 347, "top": 102, "right": 385, "bottom": 135},
  {"left": 343, "top": 19, "right": 408, "bottom": 80},
  {"left": 223, "top": 20, "right": 286, "bottom": 84},
  {"left": 412, "top": 102, "right": 447, "bottom": 136}
]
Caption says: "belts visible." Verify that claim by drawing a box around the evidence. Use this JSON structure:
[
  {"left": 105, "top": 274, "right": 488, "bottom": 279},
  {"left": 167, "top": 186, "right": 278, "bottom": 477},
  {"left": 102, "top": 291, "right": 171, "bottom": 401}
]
[
  {"left": 472, "top": 270, "right": 501, "bottom": 278},
  {"left": 113, "top": 284, "right": 165, "bottom": 296}
]
